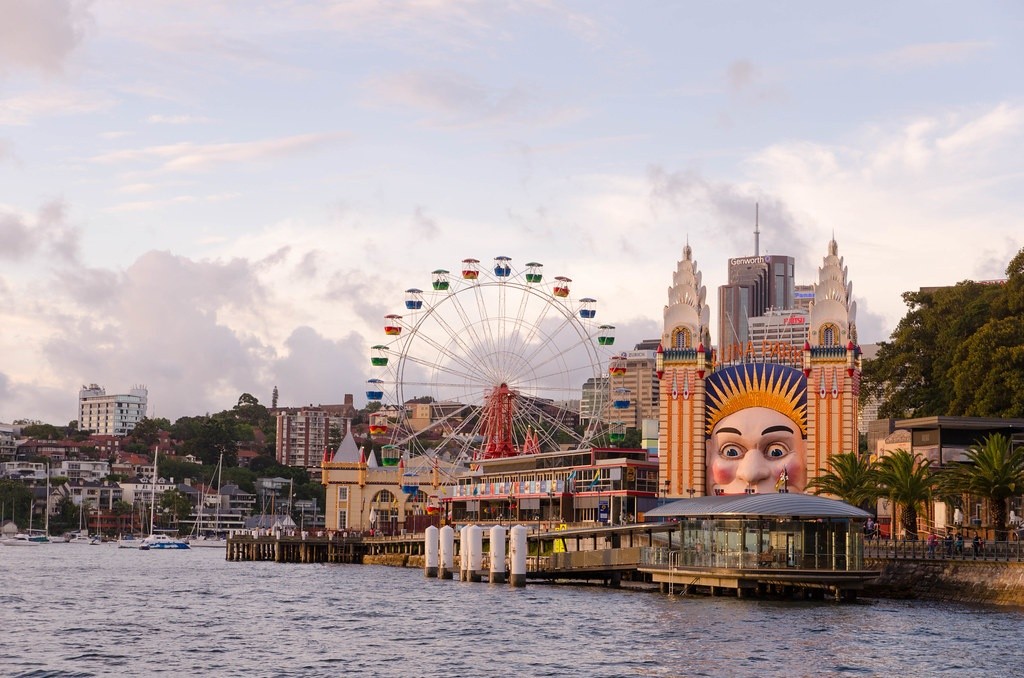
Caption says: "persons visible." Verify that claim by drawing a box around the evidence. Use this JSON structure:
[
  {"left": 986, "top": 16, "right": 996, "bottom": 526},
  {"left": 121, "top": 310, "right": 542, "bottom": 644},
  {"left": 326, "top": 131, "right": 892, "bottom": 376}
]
[
  {"left": 971, "top": 531, "right": 981, "bottom": 562},
  {"left": 247, "top": 525, "right": 383, "bottom": 539},
  {"left": 926, "top": 529, "right": 938, "bottom": 559},
  {"left": 944, "top": 531, "right": 964, "bottom": 560},
  {"left": 619, "top": 511, "right": 635, "bottom": 525}
]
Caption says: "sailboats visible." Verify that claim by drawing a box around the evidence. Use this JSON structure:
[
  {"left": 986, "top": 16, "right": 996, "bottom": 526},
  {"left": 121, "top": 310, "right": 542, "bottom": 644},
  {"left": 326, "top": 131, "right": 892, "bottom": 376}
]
[
  {"left": 3, "top": 462, "right": 102, "bottom": 546},
  {"left": 139, "top": 544, "right": 151, "bottom": 550},
  {"left": 108, "top": 444, "right": 227, "bottom": 551}
]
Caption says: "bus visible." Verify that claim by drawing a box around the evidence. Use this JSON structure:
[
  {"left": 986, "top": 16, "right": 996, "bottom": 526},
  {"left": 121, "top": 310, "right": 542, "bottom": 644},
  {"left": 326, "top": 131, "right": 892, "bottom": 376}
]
[{"left": 872, "top": 516, "right": 900, "bottom": 537}]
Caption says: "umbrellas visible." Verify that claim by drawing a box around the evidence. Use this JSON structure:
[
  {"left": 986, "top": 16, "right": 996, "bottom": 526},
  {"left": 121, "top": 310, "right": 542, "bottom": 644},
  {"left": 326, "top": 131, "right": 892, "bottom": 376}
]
[{"left": 368, "top": 506, "right": 377, "bottom": 529}]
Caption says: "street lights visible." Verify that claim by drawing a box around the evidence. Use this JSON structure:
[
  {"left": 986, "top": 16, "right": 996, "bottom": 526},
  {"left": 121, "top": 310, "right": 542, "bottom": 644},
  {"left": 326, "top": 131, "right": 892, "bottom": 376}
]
[
  {"left": 412, "top": 504, "right": 419, "bottom": 537},
  {"left": 392, "top": 507, "right": 398, "bottom": 538},
  {"left": 571, "top": 489, "right": 579, "bottom": 523},
  {"left": 472, "top": 499, "right": 478, "bottom": 521},
  {"left": 661, "top": 480, "right": 671, "bottom": 524},
  {"left": 508, "top": 495, "right": 515, "bottom": 529},
  {"left": 596, "top": 488, "right": 603, "bottom": 522},
  {"left": 547, "top": 493, "right": 555, "bottom": 529},
  {"left": 686, "top": 489, "right": 695, "bottom": 498}
]
